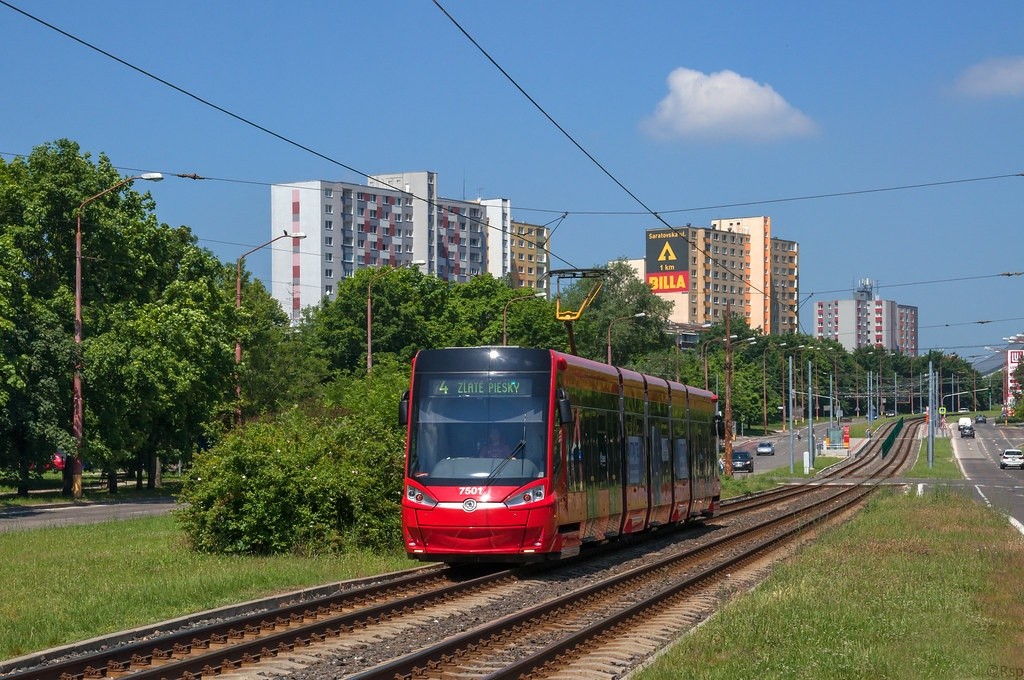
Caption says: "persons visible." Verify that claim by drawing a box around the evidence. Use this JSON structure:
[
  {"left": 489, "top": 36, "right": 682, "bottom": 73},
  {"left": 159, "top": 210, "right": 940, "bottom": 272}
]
[
  {"left": 864, "top": 427, "right": 872, "bottom": 438},
  {"left": 479, "top": 427, "right": 515, "bottom": 458},
  {"left": 797, "top": 427, "right": 803, "bottom": 441},
  {"left": 812, "top": 425, "right": 817, "bottom": 440}
]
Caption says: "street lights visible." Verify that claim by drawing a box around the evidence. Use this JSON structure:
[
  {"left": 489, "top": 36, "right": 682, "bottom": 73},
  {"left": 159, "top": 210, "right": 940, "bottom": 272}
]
[
  {"left": 366, "top": 258, "right": 428, "bottom": 374},
  {"left": 856, "top": 351, "right": 874, "bottom": 417},
  {"left": 880, "top": 353, "right": 895, "bottom": 414},
  {"left": 676, "top": 323, "right": 712, "bottom": 383},
  {"left": 725, "top": 338, "right": 756, "bottom": 476},
  {"left": 745, "top": 342, "right": 756, "bottom": 349},
  {"left": 234, "top": 232, "right": 309, "bottom": 426},
  {"left": 910, "top": 355, "right": 926, "bottom": 413},
  {"left": 607, "top": 313, "right": 646, "bottom": 365},
  {"left": 71, "top": 173, "right": 165, "bottom": 496},
  {"left": 939, "top": 354, "right": 957, "bottom": 406},
  {"left": 502, "top": 292, "right": 550, "bottom": 346},
  {"left": 705, "top": 335, "right": 737, "bottom": 391},
  {"left": 974, "top": 356, "right": 988, "bottom": 411},
  {"left": 899, "top": 354, "right": 914, "bottom": 362},
  {"left": 763, "top": 342, "right": 788, "bottom": 436},
  {"left": 782, "top": 346, "right": 833, "bottom": 426},
  {"left": 700, "top": 339, "right": 727, "bottom": 365},
  {"left": 991, "top": 334, "right": 1024, "bottom": 420}
]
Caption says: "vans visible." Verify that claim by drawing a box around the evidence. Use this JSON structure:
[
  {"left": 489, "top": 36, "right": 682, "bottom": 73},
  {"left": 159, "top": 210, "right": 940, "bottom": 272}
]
[{"left": 957, "top": 417, "right": 973, "bottom": 431}]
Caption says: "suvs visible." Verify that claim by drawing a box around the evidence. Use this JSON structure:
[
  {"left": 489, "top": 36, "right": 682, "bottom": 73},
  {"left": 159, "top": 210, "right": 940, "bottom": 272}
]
[
  {"left": 731, "top": 451, "right": 754, "bottom": 473},
  {"left": 999, "top": 449, "right": 1024, "bottom": 469},
  {"left": 975, "top": 415, "right": 987, "bottom": 424},
  {"left": 961, "top": 427, "right": 975, "bottom": 438}
]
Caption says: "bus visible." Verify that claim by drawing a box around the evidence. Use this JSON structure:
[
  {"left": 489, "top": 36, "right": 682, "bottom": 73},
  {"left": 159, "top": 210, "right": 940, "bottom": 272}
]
[{"left": 397, "top": 347, "right": 720, "bottom": 567}]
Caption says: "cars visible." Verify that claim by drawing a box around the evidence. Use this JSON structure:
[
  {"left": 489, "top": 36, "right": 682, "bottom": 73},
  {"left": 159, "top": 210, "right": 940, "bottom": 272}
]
[
  {"left": 865, "top": 411, "right": 877, "bottom": 420},
  {"left": 755, "top": 441, "right": 775, "bottom": 456},
  {"left": 959, "top": 408, "right": 969, "bottom": 413},
  {"left": 29, "top": 453, "right": 65, "bottom": 475},
  {"left": 886, "top": 409, "right": 899, "bottom": 417}
]
